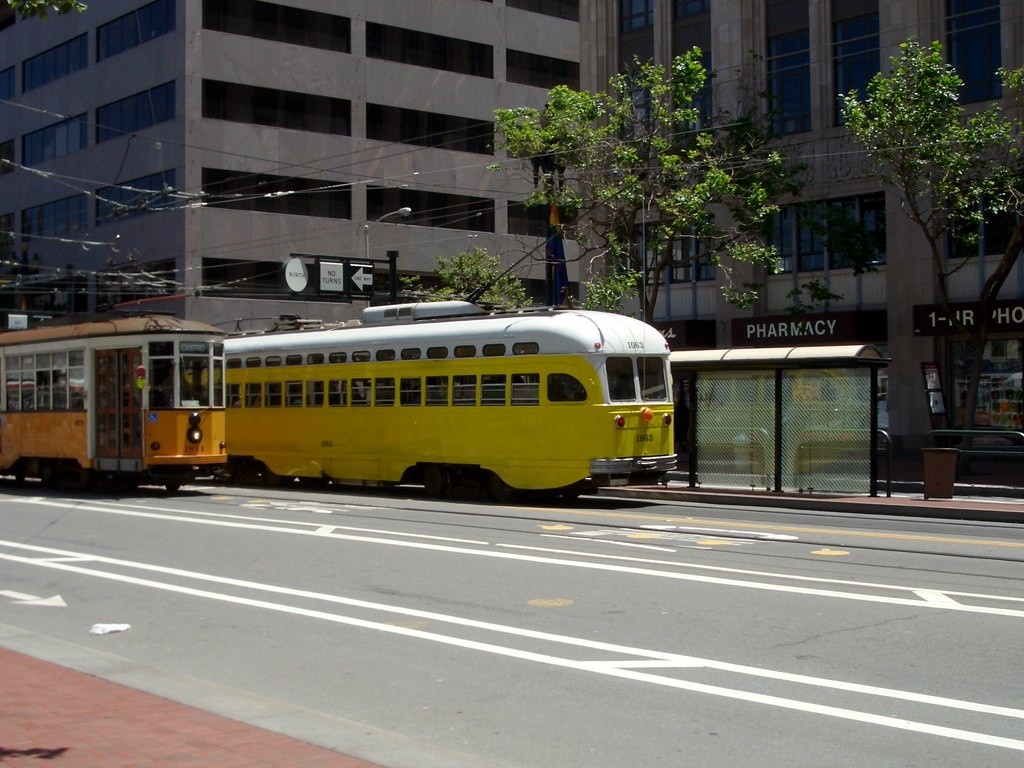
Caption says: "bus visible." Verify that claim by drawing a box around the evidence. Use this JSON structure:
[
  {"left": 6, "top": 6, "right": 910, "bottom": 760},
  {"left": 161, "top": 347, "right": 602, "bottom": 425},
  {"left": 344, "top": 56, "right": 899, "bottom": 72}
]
[
  {"left": 699, "top": 371, "right": 870, "bottom": 474},
  {"left": 137, "top": 301, "right": 679, "bottom": 507},
  {"left": 0, "top": 316, "right": 228, "bottom": 493},
  {"left": 7, "top": 381, "right": 85, "bottom": 410}
]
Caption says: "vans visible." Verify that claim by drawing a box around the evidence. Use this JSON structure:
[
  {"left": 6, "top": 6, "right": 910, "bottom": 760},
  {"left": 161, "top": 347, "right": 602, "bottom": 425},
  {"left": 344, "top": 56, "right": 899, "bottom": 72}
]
[{"left": 985, "top": 373, "right": 1023, "bottom": 421}]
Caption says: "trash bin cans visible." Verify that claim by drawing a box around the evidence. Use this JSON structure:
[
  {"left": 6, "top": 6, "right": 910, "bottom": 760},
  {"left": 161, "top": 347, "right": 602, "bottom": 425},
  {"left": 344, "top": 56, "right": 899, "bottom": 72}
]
[{"left": 921, "top": 447, "right": 960, "bottom": 500}]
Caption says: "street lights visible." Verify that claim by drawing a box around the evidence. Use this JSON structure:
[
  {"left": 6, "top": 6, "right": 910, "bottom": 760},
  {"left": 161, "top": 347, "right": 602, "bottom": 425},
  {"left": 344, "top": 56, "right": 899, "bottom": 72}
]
[{"left": 365, "top": 208, "right": 411, "bottom": 264}]
